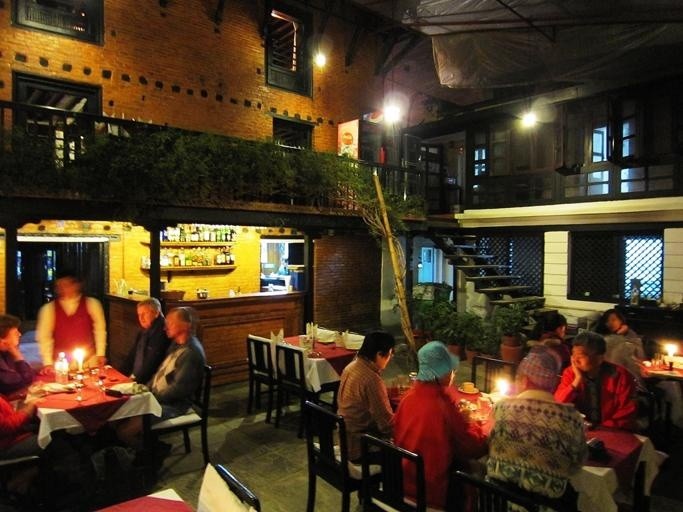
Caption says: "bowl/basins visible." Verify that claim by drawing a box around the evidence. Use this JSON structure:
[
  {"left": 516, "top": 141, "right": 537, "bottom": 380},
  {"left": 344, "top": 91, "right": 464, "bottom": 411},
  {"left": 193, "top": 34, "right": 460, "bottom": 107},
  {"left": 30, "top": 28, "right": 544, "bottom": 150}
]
[{"left": 196, "top": 292, "right": 207, "bottom": 299}]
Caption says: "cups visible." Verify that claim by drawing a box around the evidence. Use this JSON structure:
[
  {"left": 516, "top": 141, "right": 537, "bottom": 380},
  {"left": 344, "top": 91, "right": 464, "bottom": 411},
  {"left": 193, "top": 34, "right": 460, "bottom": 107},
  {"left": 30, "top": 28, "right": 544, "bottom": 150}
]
[
  {"left": 299, "top": 334, "right": 313, "bottom": 352},
  {"left": 462, "top": 382, "right": 474, "bottom": 391}
]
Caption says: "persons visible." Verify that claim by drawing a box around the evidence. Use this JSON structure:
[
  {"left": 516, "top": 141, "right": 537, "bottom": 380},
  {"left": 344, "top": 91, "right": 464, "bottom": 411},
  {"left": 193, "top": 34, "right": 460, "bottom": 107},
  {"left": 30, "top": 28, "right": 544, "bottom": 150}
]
[
  {"left": 338, "top": 310, "right": 650, "bottom": 512},
  {"left": 0, "top": 268, "right": 206, "bottom": 494}
]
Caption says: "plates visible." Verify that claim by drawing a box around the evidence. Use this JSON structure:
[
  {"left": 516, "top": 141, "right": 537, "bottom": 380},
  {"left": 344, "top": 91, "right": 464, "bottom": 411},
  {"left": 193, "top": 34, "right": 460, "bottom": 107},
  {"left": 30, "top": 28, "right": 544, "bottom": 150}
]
[{"left": 458, "top": 386, "right": 480, "bottom": 394}]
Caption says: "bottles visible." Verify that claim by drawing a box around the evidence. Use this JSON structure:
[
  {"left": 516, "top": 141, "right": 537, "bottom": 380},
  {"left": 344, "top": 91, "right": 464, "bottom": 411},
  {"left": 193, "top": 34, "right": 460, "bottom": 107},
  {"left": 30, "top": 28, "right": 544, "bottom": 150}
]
[
  {"left": 631, "top": 287, "right": 640, "bottom": 306},
  {"left": 161, "top": 224, "right": 234, "bottom": 267},
  {"left": 53, "top": 352, "right": 69, "bottom": 384}
]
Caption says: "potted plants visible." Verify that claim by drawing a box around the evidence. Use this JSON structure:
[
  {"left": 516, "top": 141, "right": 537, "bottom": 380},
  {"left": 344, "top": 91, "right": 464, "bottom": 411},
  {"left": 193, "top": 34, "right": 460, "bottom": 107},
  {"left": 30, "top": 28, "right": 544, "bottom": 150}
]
[{"left": 417, "top": 302, "right": 532, "bottom": 372}]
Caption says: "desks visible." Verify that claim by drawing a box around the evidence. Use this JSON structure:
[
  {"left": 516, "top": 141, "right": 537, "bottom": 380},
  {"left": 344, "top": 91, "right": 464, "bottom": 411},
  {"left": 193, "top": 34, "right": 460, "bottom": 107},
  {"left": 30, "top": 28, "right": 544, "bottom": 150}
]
[
  {"left": 377, "top": 364, "right": 670, "bottom": 511},
  {"left": 635, "top": 353, "right": 682, "bottom": 444},
  {"left": 269, "top": 333, "right": 361, "bottom": 418}
]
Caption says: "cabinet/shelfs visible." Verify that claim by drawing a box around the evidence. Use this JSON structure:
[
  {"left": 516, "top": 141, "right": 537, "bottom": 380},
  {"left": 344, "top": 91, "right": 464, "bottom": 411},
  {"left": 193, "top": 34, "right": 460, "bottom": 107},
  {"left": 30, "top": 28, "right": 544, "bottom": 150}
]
[{"left": 141, "top": 241, "right": 236, "bottom": 282}]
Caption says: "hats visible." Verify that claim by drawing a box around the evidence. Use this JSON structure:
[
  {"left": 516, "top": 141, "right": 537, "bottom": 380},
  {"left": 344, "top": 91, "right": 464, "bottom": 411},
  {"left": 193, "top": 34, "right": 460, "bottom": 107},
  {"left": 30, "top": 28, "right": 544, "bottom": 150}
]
[
  {"left": 415, "top": 341, "right": 459, "bottom": 381},
  {"left": 518, "top": 345, "right": 558, "bottom": 386}
]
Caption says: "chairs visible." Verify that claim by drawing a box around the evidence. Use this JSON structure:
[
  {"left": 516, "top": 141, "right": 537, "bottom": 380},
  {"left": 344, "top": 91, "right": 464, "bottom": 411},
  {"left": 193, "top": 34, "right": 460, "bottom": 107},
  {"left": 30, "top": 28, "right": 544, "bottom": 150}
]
[
  {"left": 208, "top": 464, "right": 265, "bottom": 512},
  {"left": 274, "top": 341, "right": 341, "bottom": 438},
  {"left": 471, "top": 355, "right": 517, "bottom": 401},
  {"left": 148, "top": 363, "right": 213, "bottom": 467},
  {"left": 0, "top": 454, "right": 42, "bottom": 512},
  {"left": 302, "top": 397, "right": 384, "bottom": 512},
  {"left": 447, "top": 467, "right": 541, "bottom": 512},
  {"left": 239, "top": 334, "right": 275, "bottom": 430},
  {"left": 356, "top": 429, "right": 427, "bottom": 512},
  {"left": 635, "top": 390, "right": 657, "bottom": 448}
]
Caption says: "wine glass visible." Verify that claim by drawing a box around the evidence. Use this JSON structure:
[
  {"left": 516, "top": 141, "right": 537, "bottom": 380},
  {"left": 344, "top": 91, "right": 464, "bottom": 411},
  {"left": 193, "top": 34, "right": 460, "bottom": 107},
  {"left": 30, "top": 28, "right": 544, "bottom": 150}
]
[{"left": 73, "top": 375, "right": 87, "bottom": 401}]
